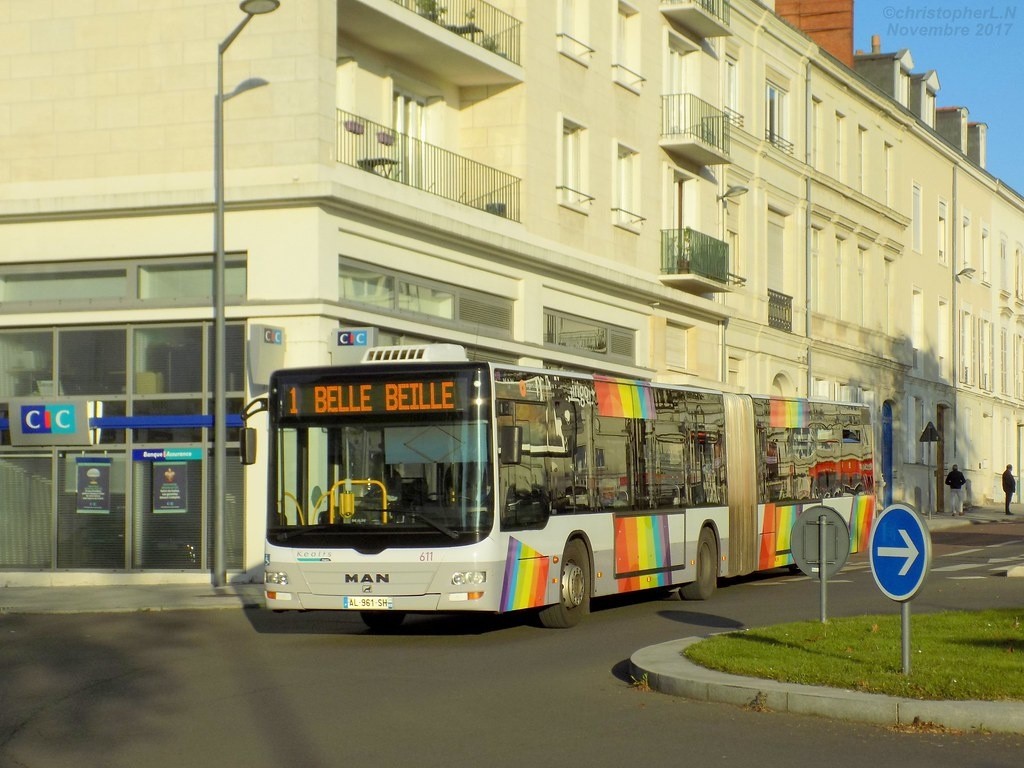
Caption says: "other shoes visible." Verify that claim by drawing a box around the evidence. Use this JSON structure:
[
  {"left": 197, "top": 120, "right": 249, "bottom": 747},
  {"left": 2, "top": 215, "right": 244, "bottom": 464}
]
[
  {"left": 951, "top": 513, "right": 955, "bottom": 516},
  {"left": 1005, "top": 512, "right": 1014, "bottom": 515},
  {"left": 958, "top": 512, "right": 963, "bottom": 516}
]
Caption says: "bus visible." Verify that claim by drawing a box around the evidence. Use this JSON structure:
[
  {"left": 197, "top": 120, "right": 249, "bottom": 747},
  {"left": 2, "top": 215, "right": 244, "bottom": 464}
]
[{"left": 238, "top": 343, "right": 890, "bottom": 630}]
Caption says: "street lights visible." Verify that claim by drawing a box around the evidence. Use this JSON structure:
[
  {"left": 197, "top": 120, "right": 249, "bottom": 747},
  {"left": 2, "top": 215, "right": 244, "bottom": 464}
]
[{"left": 209, "top": 0, "right": 282, "bottom": 589}]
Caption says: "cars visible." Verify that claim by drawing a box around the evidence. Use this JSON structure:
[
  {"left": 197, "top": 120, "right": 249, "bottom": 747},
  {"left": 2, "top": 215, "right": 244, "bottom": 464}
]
[{"left": 564, "top": 483, "right": 600, "bottom": 507}]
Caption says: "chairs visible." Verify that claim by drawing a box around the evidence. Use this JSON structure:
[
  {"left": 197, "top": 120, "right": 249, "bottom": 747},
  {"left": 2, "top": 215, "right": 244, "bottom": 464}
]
[{"left": 360, "top": 442, "right": 874, "bottom": 521}]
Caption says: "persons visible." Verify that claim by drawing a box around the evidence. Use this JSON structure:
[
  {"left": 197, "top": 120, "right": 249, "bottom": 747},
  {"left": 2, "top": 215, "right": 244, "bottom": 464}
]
[
  {"left": 597, "top": 479, "right": 627, "bottom": 507},
  {"left": 944, "top": 464, "right": 967, "bottom": 516},
  {"left": 1002, "top": 464, "right": 1016, "bottom": 515}
]
